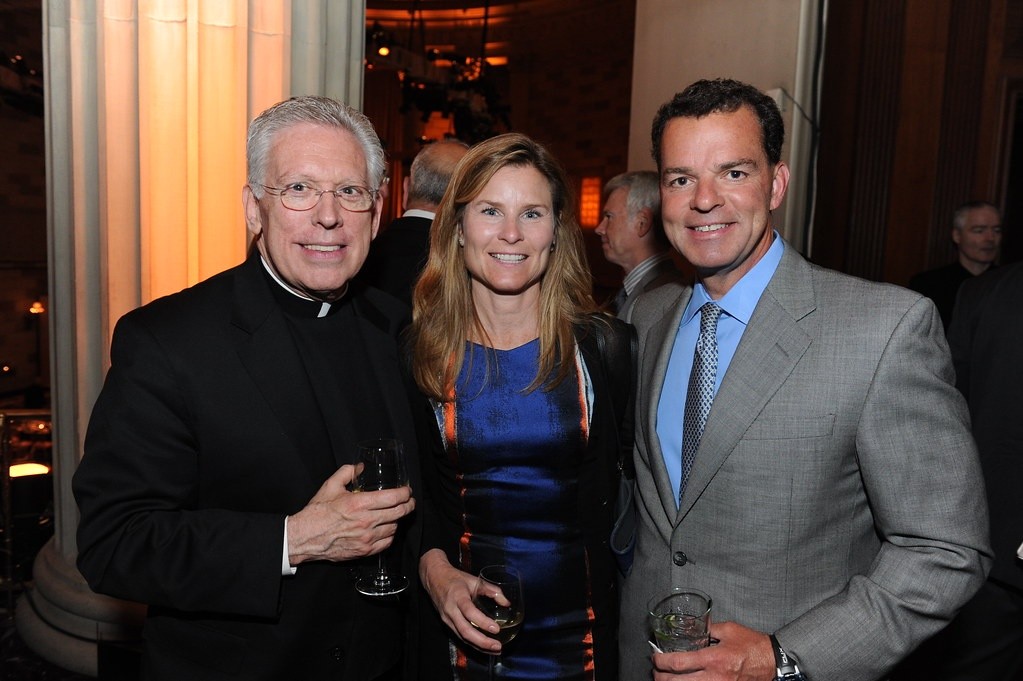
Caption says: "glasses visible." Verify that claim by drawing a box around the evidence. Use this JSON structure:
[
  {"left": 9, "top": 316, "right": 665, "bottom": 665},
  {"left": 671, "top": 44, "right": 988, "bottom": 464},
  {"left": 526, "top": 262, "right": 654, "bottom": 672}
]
[{"left": 252, "top": 180, "right": 379, "bottom": 213}]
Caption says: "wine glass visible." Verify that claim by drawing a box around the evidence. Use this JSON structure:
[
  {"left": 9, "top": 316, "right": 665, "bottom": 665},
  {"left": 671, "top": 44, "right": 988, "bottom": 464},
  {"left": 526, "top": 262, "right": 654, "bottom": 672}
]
[
  {"left": 351, "top": 438, "right": 410, "bottom": 596},
  {"left": 469, "top": 565, "right": 525, "bottom": 681}
]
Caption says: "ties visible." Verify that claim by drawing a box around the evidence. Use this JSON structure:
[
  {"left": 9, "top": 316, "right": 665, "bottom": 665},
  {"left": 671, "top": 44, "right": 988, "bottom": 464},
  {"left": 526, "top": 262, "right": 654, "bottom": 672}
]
[
  {"left": 616, "top": 287, "right": 627, "bottom": 312},
  {"left": 675, "top": 302, "right": 724, "bottom": 509}
]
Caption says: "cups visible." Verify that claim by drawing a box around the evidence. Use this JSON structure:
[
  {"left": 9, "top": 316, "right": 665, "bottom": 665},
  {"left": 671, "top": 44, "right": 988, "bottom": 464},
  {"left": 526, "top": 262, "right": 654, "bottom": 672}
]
[{"left": 647, "top": 587, "right": 714, "bottom": 652}]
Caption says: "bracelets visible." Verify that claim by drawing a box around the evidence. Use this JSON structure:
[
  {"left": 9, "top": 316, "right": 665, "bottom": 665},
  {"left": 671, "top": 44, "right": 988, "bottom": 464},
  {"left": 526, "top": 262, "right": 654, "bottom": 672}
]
[{"left": 623, "top": 80, "right": 996, "bottom": 681}]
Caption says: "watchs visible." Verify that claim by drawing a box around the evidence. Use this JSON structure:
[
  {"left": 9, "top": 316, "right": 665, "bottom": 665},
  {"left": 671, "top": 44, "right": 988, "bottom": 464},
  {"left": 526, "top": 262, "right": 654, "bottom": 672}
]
[{"left": 770, "top": 632, "right": 806, "bottom": 681}]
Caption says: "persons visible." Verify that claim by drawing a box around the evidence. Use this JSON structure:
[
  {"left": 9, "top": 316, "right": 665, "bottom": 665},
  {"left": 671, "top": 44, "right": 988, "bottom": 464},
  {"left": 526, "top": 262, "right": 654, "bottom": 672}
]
[
  {"left": 396, "top": 132, "right": 639, "bottom": 681},
  {"left": 889, "top": 262, "right": 1023, "bottom": 680},
  {"left": 369, "top": 139, "right": 470, "bottom": 303},
  {"left": 595, "top": 171, "right": 689, "bottom": 322},
  {"left": 912, "top": 202, "right": 1003, "bottom": 334},
  {"left": 70, "top": 97, "right": 424, "bottom": 681}
]
